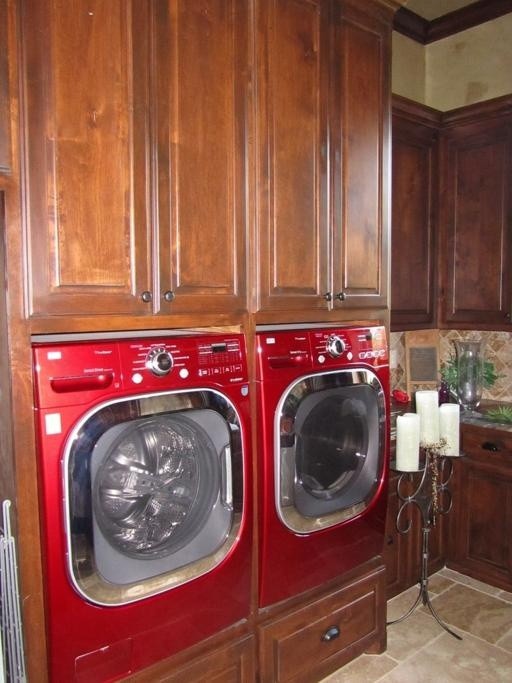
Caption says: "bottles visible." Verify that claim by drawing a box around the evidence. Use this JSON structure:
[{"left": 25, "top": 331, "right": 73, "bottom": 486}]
[{"left": 438, "top": 382, "right": 449, "bottom": 404}]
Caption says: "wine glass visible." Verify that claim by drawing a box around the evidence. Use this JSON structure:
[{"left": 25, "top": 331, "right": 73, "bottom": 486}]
[{"left": 452, "top": 337, "right": 483, "bottom": 423}]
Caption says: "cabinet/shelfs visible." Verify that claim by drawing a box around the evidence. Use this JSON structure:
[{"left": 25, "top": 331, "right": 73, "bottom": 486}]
[
  {"left": 447, "top": 416, "right": 512, "bottom": 593},
  {"left": 116, "top": 616, "right": 261, "bottom": 682},
  {"left": 259, "top": 562, "right": 387, "bottom": 679},
  {"left": 4, "top": 0, "right": 244, "bottom": 328},
  {"left": 387, "top": 427, "right": 446, "bottom": 598},
  {"left": 393, "top": 92, "right": 438, "bottom": 334},
  {"left": 245, "top": 0, "right": 392, "bottom": 327},
  {"left": 438, "top": 93, "right": 512, "bottom": 334}
]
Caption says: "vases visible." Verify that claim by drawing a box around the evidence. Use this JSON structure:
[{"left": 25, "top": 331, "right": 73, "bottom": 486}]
[{"left": 448, "top": 335, "right": 487, "bottom": 407}]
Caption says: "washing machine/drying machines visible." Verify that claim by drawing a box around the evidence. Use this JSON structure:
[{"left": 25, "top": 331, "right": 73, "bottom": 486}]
[
  {"left": 27, "top": 330, "right": 255, "bottom": 683},
  {"left": 253, "top": 325, "right": 393, "bottom": 611}
]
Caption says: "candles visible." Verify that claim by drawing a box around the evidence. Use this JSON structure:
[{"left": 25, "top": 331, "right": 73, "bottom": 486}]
[{"left": 391, "top": 390, "right": 459, "bottom": 470}]
